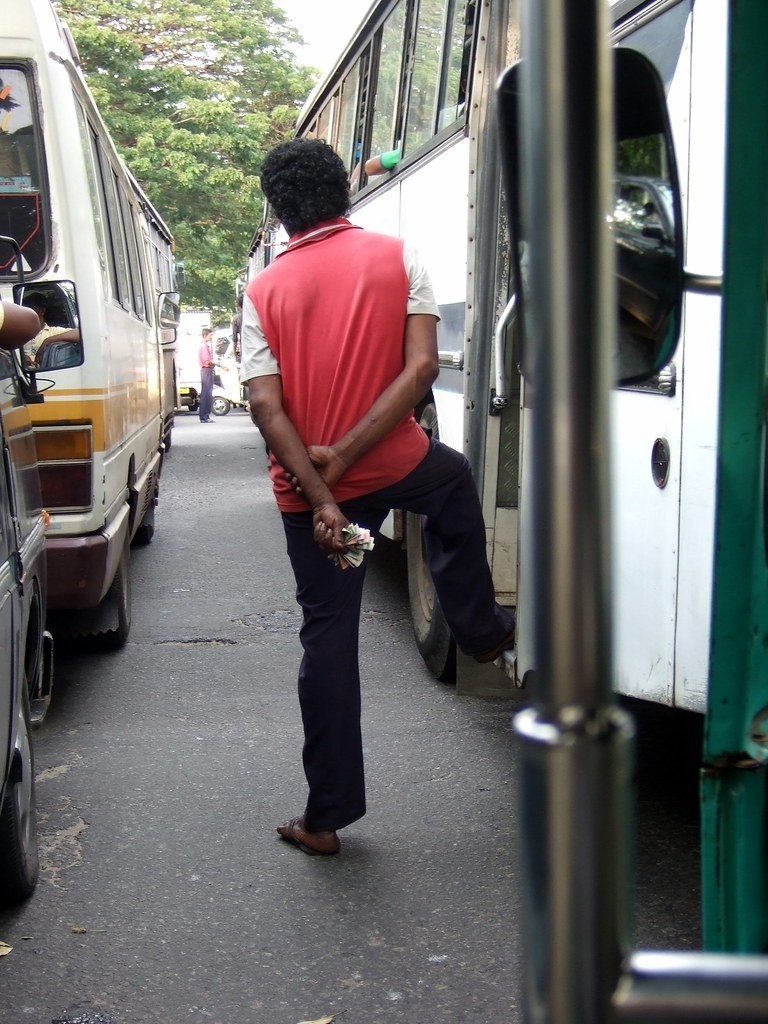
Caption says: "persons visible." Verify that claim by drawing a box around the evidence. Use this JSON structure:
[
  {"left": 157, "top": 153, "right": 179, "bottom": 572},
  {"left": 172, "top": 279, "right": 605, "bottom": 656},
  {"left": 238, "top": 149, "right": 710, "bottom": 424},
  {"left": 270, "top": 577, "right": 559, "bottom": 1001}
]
[
  {"left": 348, "top": 65, "right": 468, "bottom": 197},
  {"left": 0, "top": 301, "right": 40, "bottom": 351},
  {"left": 22, "top": 293, "right": 81, "bottom": 369},
  {"left": 231, "top": 295, "right": 243, "bottom": 362},
  {"left": 198, "top": 328, "right": 216, "bottom": 424},
  {"left": 238, "top": 138, "right": 516, "bottom": 859}
]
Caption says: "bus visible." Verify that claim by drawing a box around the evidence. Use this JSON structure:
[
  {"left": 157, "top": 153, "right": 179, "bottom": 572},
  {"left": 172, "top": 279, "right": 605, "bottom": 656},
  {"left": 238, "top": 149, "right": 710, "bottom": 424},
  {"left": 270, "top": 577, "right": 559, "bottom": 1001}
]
[
  {"left": 0, "top": 1, "right": 182, "bottom": 660},
  {"left": 225, "top": 0, "right": 728, "bottom": 798}
]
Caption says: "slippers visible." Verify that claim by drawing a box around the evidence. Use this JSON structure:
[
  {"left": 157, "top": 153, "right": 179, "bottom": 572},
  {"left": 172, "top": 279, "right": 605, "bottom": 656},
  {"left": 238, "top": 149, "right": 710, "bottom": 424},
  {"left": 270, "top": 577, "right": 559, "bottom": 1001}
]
[
  {"left": 471, "top": 610, "right": 517, "bottom": 665},
  {"left": 284, "top": 818, "right": 325, "bottom": 857}
]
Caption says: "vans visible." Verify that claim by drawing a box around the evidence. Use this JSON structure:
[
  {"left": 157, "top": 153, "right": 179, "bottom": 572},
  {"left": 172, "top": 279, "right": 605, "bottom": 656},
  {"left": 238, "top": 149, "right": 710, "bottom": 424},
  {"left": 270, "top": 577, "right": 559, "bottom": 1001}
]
[{"left": 0, "top": 285, "right": 58, "bottom": 930}]
